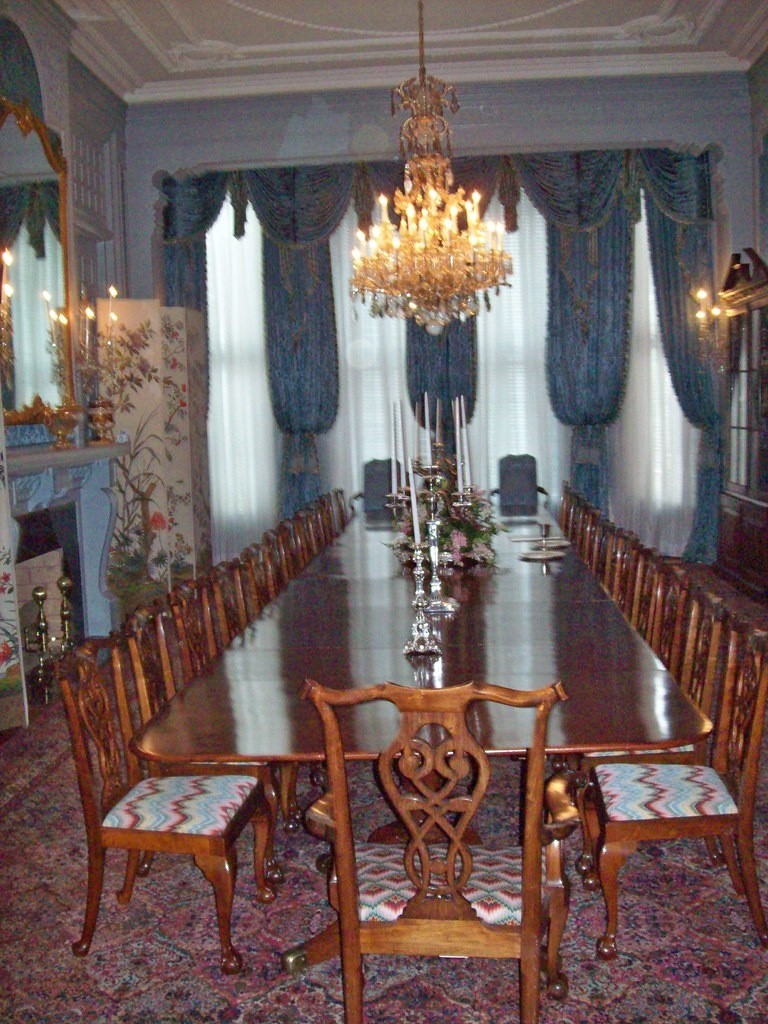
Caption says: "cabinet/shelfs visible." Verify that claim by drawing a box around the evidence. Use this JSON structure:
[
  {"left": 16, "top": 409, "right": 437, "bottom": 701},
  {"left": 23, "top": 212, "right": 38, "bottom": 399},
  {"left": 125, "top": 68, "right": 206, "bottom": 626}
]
[{"left": 717, "top": 247, "right": 768, "bottom": 607}]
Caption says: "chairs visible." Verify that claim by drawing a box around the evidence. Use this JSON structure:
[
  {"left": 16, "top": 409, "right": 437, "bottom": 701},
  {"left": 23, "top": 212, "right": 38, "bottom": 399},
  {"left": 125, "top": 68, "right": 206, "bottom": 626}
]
[
  {"left": 57, "top": 487, "right": 344, "bottom": 974},
  {"left": 488, "top": 454, "right": 549, "bottom": 505},
  {"left": 557, "top": 480, "right": 768, "bottom": 960},
  {"left": 299, "top": 677, "right": 581, "bottom": 1024},
  {"left": 348, "top": 459, "right": 411, "bottom": 518}
]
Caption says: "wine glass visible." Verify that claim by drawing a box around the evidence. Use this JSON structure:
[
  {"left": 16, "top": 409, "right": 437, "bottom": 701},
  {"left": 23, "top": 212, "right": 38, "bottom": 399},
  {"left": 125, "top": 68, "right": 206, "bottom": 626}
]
[{"left": 539, "top": 523, "right": 550, "bottom": 550}]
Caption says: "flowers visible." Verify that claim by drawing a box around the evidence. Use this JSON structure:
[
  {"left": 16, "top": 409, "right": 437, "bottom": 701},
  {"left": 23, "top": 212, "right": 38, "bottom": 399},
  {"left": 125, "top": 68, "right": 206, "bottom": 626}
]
[{"left": 384, "top": 483, "right": 510, "bottom": 580}]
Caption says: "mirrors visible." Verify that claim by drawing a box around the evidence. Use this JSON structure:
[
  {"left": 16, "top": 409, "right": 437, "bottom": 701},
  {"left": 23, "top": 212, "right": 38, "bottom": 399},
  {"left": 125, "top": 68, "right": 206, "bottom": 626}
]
[{"left": 1, "top": 95, "right": 79, "bottom": 427}]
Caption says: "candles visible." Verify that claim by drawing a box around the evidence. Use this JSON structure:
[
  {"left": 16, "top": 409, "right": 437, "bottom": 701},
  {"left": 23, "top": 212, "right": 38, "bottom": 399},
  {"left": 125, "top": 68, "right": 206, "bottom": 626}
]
[{"left": 392, "top": 392, "right": 471, "bottom": 545}]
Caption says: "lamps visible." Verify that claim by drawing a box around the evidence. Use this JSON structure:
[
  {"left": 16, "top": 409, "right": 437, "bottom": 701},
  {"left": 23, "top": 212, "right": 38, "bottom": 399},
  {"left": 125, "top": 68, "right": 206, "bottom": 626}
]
[
  {"left": 1, "top": 245, "right": 15, "bottom": 390},
  {"left": 80, "top": 286, "right": 118, "bottom": 388},
  {"left": 41, "top": 290, "right": 69, "bottom": 403},
  {"left": 350, "top": 0, "right": 515, "bottom": 337}
]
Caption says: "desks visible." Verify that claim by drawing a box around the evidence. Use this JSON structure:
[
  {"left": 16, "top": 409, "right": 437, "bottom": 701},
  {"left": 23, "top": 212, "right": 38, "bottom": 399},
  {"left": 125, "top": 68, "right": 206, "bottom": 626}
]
[{"left": 130, "top": 504, "right": 715, "bottom": 846}]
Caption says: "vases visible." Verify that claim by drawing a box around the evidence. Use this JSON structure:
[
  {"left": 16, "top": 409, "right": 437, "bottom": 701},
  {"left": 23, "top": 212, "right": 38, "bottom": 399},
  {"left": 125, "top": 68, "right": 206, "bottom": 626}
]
[{"left": 405, "top": 557, "right": 476, "bottom": 571}]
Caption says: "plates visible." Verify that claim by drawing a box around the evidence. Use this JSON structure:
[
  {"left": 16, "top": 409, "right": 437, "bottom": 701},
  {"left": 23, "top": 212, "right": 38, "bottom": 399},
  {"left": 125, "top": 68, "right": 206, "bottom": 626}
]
[
  {"left": 519, "top": 551, "right": 566, "bottom": 561},
  {"left": 534, "top": 540, "right": 570, "bottom": 548}
]
